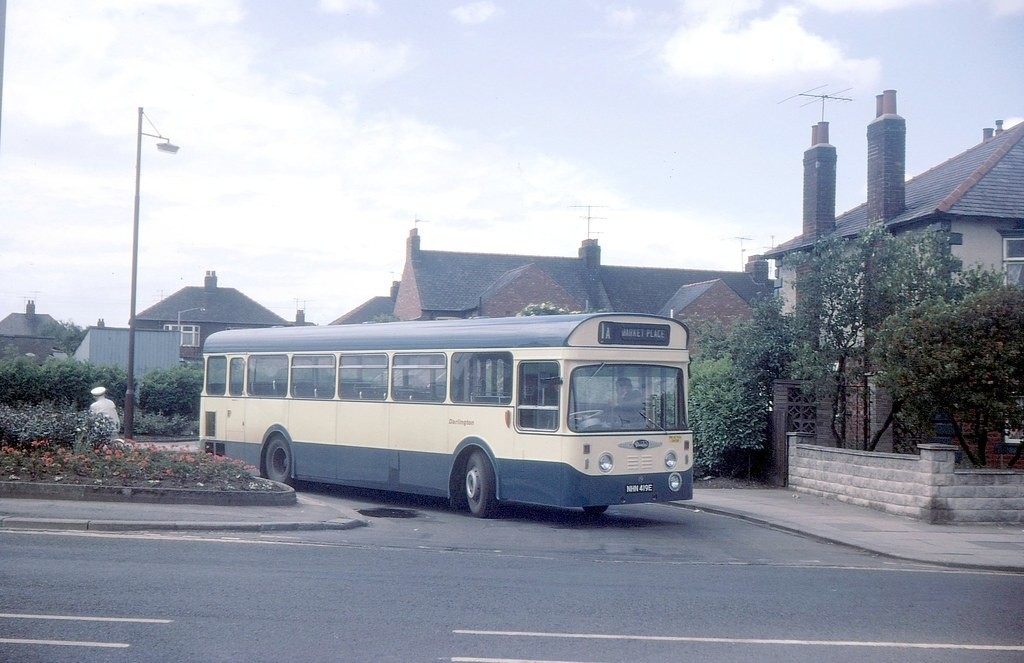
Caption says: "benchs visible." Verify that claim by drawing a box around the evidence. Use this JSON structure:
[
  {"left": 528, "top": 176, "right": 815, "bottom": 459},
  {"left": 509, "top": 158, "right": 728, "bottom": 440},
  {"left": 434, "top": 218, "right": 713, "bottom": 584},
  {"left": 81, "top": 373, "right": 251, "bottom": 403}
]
[{"left": 209, "top": 377, "right": 512, "bottom": 404}]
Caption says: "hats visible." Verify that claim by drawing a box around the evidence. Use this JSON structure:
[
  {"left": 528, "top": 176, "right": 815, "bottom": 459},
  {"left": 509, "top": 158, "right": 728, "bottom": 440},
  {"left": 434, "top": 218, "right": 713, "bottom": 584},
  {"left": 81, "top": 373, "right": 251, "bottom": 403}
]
[{"left": 91, "top": 387, "right": 106, "bottom": 397}]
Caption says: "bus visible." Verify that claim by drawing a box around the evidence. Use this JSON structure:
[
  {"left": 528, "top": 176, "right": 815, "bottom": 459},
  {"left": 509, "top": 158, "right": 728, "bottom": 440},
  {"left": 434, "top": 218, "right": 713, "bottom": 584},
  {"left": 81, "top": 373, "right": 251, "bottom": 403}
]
[{"left": 197, "top": 310, "right": 700, "bottom": 520}]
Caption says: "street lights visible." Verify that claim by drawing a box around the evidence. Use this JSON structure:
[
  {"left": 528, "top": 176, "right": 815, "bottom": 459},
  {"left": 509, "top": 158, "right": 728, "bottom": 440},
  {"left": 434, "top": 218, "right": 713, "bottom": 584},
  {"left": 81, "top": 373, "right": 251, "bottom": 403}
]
[{"left": 124, "top": 102, "right": 181, "bottom": 449}]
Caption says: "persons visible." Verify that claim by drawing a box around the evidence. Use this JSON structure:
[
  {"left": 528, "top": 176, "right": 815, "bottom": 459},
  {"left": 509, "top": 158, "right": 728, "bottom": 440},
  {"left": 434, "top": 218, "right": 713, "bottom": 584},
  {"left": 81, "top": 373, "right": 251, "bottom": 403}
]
[
  {"left": 89, "top": 386, "right": 120, "bottom": 436},
  {"left": 609, "top": 378, "right": 645, "bottom": 430}
]
